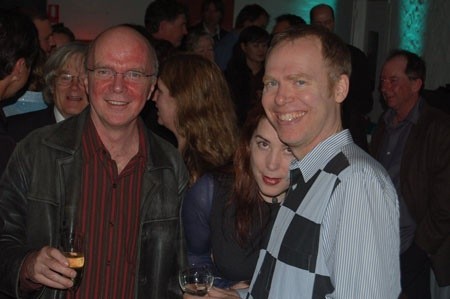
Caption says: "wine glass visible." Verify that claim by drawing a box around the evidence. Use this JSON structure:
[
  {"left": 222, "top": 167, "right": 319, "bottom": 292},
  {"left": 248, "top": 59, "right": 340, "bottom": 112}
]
[{"left": 58, "top": 231, "right": 85, "bottom": 299}]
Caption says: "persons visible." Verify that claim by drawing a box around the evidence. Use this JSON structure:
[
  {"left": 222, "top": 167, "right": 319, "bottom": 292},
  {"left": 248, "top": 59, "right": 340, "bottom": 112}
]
[
  {"left": 0, "top": 0, "right": 450, "bottom": 299},
  {"left": 182, "top": 21, "right": 402, "bottom": 299}
]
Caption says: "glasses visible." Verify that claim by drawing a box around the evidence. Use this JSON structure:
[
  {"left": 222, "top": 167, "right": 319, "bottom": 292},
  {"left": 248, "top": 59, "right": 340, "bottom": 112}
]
[
  {"left": 88, "top": 67, "right": 156, "bottom": 85},
  {"left": 53, "top": 71, "right": 89, "bottom": 86}
]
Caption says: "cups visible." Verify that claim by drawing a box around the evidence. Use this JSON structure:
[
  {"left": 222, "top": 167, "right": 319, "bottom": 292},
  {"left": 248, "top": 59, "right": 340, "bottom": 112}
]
[{"left": 178, "top": 256, "right": 214, "bottom": 297}]
